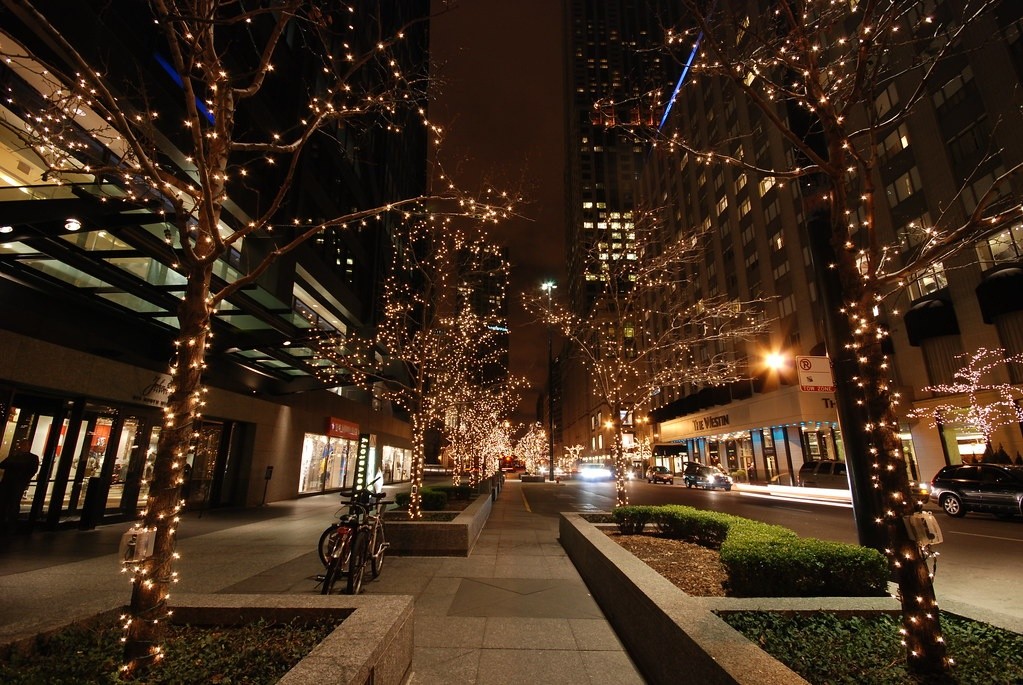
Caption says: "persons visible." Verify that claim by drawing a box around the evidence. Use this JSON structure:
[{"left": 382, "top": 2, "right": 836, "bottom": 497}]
[{"left": 0, "top": 438, "right": 40, "bottom": 529}]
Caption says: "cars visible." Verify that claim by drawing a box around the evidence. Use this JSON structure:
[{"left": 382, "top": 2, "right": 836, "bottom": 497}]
[
  {"left": 538, "top": 465, "right": 570, "bottom": 480},
  {"left": 909, "top": 481, "right": 929, "bottom": 509}
]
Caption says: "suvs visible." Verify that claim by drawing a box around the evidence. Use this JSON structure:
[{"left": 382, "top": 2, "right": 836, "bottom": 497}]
[
  {"left": 646, "top": 466, "right": 674, "bottom": 485},
  {"left": 798, "top": 460, "right": 849, "bottom": 489},
  {"left": 683, "top": 461, "right": 733, "bottom": 491},
  {"left": 571, "top": 463, "right": 610, "bottom": 482},
  {"left": 929, "top": 463, "right": 1023, "bottom": 520}
]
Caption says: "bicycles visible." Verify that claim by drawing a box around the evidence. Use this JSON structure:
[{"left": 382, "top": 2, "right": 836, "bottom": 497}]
[{"left": 318, "top": 486, "right": 394, "bottom": 596}]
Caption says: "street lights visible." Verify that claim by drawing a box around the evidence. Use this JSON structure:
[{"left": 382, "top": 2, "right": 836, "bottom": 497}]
[
  {"left": 636, "top": 416, "right": 648, "bottom": 480},
  {"left": 542, "top": 281, "right": 557, "bottom": 481},
  {"left": 606, "top": 421, "right": 613, "bottom": 461}
]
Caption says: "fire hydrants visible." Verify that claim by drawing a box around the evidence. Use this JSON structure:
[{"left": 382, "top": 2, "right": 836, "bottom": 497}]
[{"left": 554, "top": 476, "right": 560, "bottom": 484}]
